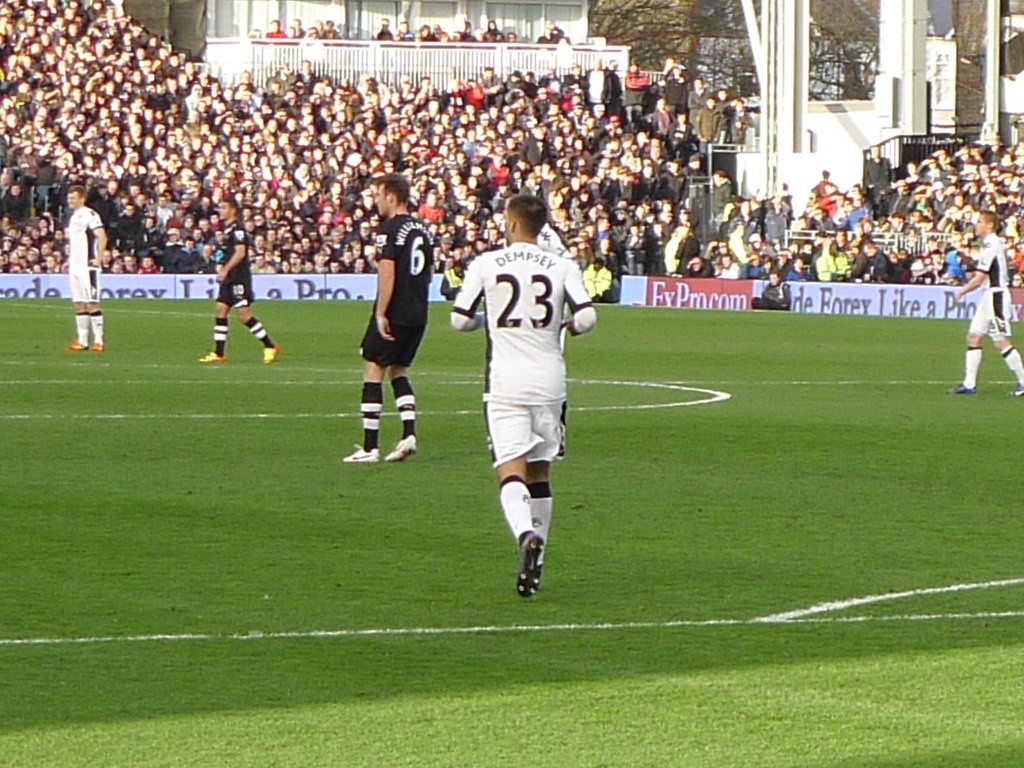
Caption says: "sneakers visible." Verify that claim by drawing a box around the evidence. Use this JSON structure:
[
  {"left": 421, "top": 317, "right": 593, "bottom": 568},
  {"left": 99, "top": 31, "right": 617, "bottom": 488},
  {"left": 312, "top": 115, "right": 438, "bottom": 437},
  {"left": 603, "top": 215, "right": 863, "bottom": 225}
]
[
  {"left": 198, "top": 351, "right": 227, "bottom": 362},
  {"left": 1008, "top": 384, "right": 1023, "bottom": 397},
  {"left": 517, "top": 533, "right": 545, "bottom": 598},
  {"left": 264, "top": 346, "right": 280, "bottom": 365},
  {"left": 385, "top": 435, "right": 417, "bottom": 462},
  {"left": 91, "top": 342, "right": 103, "bottom": 351},
  {"left": 67, "top": 341, "right": 89, "bottom": 351},
  {"left": 945, "top": 384, "right": 977, "bottom": 396},
  {"left": 343, "top": 443, "right": 380, "bottom": 462}
]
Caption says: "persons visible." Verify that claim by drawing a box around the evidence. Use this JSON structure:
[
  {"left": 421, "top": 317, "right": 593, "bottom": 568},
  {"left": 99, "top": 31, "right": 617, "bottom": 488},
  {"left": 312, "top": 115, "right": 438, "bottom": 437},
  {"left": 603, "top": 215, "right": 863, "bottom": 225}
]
[
  {"left": 751, "top": 271, "right": 791, "bottom": 311},
  {"left": 342, "top": 174, "right": 434, "bottom": 463},
  {"left": 66, "top": 185, "right": 108, "bottom": 352},
  {"left": 198, "top": 199, "right": 279, "bottom": 364},
  {"left": 0, "top": 0, "right": 1024, "bottom": 304},
  {"left": 946, "top": 210, "right": 1024, "bottom": 397},
  {"left": 450, "top": 196, "right": 596, "bottom": 597}
]
